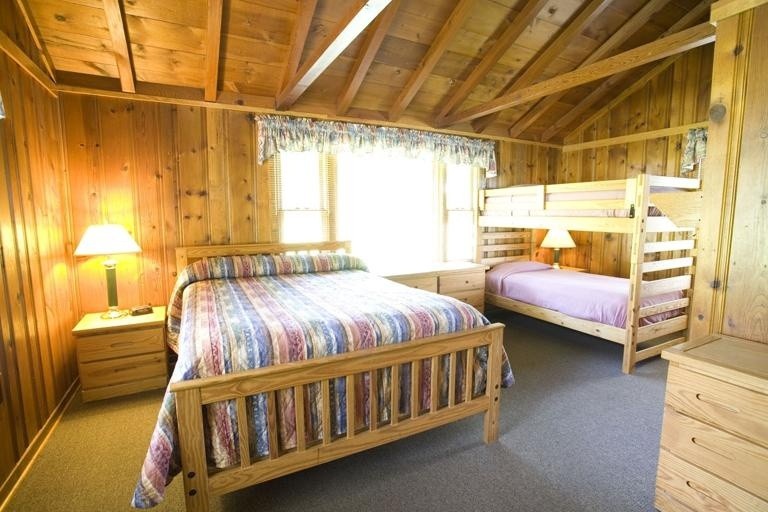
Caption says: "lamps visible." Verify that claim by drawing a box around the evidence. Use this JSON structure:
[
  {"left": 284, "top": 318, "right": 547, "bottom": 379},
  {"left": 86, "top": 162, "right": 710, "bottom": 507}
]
[
  {"left": 73, "top": 224, "right": 143, "bottom": 321},
  {"left": 541, "top": 229, "right": 576, "bottom": 269}
]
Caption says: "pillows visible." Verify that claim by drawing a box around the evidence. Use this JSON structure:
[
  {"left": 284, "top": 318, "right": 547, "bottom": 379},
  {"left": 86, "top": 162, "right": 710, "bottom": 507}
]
[
  {"left": 491, "top": 260, "right": 554, "bottom": 279},
  {"left": 182, "top": 252, "right": 372, "bottom": 288}
]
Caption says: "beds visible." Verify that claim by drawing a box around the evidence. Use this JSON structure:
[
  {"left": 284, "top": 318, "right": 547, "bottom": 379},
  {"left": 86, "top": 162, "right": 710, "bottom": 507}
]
[
  {"left": 168, "top": 239, "right": 506, "bottom": 512},
  {"left": 474, "top": 167, "right": 700, "bottom": 374}
]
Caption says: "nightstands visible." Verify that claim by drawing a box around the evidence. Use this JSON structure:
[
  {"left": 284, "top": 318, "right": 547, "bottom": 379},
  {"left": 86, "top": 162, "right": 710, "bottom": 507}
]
[
  {"left": 560, "top": 266, "right": 588, "bottom": 274},
  {"left": 71, "top": 304, "right": 169, "bottom": 405},
  {"left": 373, "top": 259, "right": 490, "bottom": 316}
]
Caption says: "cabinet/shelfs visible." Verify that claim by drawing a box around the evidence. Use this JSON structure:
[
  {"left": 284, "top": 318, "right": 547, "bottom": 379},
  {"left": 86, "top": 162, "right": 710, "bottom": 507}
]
[{"left": 652, "top": 332, "right": 767, "bottom": 512}]
